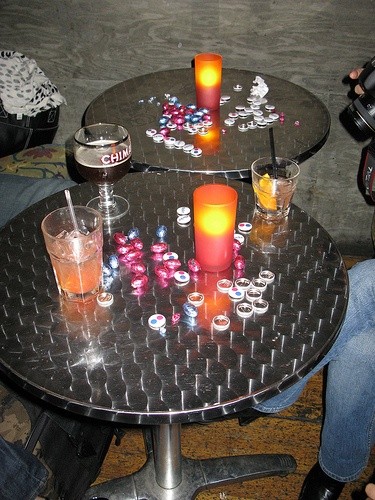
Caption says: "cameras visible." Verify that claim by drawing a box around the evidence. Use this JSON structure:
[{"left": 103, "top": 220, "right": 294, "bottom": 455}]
[{"left": 339, "top": 55, "right": 375, "bottom": 142}]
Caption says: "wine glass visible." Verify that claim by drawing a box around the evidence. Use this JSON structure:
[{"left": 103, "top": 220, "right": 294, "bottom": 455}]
[{"left": 73, "top": 123, "right": 131, "bottom": 219}]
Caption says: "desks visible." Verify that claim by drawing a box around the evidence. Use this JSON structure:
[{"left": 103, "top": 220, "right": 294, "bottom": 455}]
[
  {"left": 81, "top": 66, "right": 333, "bottom": 174},
  {"left": 0, "top": 170, "right": 350, "bottom": 499}
]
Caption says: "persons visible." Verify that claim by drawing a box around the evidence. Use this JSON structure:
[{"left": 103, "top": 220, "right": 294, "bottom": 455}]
[
  {"left": 349, "top": 68, "right": 365, "bottom": 95},
  {"left": 251, "top": 258, "right": 375, "bottom": 500}
]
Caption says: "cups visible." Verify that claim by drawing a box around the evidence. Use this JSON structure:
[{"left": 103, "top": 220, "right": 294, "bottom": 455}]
[
  {"left": 41, "top": 205, "right": 104, "bottom": 304},
  {"left": 193, "top": 184, "right": 238, "bottom": 272},
  {"left": 195, "top": 54, "right": 222, "bottom": 110},
  {"left": 251, "top": 156, "right": 301, "bottom": 221}
]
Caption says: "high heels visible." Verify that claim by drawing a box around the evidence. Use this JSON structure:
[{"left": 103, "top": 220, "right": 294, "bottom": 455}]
[
  {"left": 197, "top": 408, "right": 274, "bottom": 426},
  {"left": 297, "top": 461, "right": 345, "bottom": 500}
]
